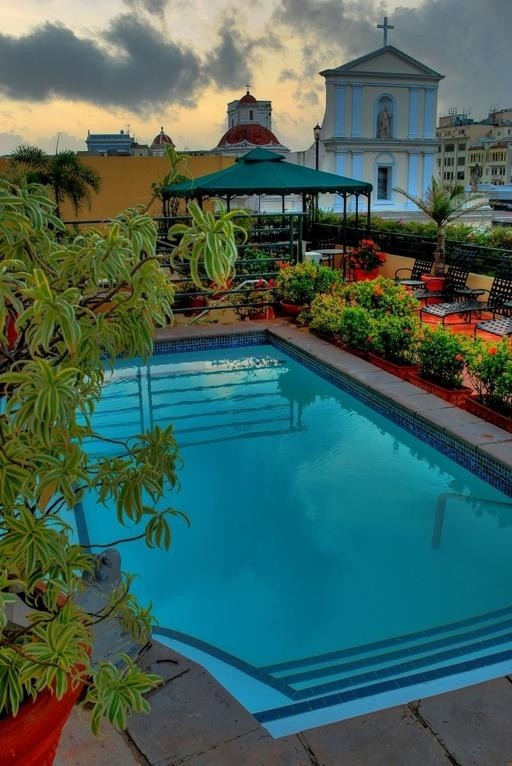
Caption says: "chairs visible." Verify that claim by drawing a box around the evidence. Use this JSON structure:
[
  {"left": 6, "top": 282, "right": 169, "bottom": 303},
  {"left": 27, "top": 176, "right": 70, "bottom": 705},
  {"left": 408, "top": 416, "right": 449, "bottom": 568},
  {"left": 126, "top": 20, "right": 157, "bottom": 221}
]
[{"left": 306, "top": 237, "right": 512, "bottom": 342}]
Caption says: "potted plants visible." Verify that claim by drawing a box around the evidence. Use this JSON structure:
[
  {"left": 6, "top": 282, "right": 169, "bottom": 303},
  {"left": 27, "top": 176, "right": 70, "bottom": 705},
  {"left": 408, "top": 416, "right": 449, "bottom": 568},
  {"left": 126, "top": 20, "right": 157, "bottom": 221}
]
[
  {"left": 0, "top": 145, "right": 249, "bottom": 766},
  {"left": 393, "top": 174, "right": 491, "bottom": 292}
]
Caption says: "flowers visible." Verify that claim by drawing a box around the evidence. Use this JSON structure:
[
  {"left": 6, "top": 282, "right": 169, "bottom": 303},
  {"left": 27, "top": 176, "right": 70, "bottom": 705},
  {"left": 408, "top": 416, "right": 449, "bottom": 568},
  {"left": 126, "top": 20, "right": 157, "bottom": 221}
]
[
  {"left": 278, "top": 262, "right": 345, "bottom": 300},
  {"left": 250, "top": 279, "right": 276, "bottom": 306},
  {"left": 311, "top": 276, "right": 512, "bottom": 416}
]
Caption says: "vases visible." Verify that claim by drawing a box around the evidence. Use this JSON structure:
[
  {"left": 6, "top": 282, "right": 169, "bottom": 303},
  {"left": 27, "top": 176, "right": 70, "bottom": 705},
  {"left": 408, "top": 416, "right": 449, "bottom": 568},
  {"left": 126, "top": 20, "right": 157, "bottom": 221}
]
[
  {"left": 279, "top": 300, "right": 306, "bottom": 315},
  {"left": 323, "top": 341, "right": 512, "bottom": 433},
  {"left": 251, "top": 306, "right": 275, "bottom": 320}
]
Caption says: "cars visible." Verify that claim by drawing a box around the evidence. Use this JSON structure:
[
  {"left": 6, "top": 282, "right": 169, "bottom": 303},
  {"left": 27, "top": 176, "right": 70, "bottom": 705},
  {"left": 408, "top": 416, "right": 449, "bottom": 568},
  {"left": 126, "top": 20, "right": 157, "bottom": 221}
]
[{"left": 491, "top": 216, "right": 512, "bottom": 227}]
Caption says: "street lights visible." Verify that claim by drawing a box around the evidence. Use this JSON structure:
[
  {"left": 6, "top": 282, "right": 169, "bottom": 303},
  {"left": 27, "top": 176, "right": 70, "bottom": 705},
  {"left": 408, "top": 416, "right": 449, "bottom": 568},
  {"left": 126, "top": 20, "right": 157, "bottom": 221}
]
[{"left": 313, "top": 122, "right": 322, "bottom": 208}]
